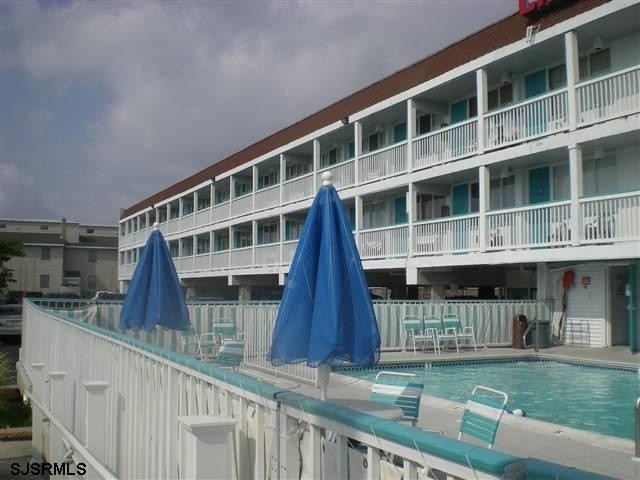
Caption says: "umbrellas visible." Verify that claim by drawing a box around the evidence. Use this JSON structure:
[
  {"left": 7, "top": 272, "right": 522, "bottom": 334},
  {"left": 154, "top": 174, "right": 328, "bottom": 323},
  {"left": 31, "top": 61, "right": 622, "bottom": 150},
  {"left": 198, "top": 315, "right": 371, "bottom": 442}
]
[
  {"left": 119, "top": 221, "right": 191, "bottom": 333},
  {"left": 264, "top": 171, "right": 381, "bottom": 401}
]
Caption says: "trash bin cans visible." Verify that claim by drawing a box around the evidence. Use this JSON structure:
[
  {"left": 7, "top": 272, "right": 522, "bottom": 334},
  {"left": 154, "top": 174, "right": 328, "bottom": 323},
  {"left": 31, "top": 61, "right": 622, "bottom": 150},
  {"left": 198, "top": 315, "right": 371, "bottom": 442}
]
[
  {"left": 513, "top": 312, "right": 528, "bottom": 351},
  {"left": 529, "top": 319, "right": 550, "bottom": 349}
]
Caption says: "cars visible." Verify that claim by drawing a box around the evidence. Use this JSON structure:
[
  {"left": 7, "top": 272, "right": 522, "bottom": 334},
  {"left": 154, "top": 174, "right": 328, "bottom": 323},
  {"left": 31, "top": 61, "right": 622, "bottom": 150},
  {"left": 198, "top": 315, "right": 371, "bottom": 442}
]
[
  {"left": 48, "top": 292, "right": 84, "bottom": 308},
  {"left": 0, "top": 289, "right": 43, "bottom": 315},
  {"left": 92, "top": 291, "right": 127, "bottom": 302}
]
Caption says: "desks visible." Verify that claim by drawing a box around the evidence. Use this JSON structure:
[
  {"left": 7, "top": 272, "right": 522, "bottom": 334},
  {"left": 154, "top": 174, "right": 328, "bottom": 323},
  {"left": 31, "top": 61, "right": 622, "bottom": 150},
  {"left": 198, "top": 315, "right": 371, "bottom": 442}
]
[{"left": 324, "top": 399, "right": 404, "bottom": 420}]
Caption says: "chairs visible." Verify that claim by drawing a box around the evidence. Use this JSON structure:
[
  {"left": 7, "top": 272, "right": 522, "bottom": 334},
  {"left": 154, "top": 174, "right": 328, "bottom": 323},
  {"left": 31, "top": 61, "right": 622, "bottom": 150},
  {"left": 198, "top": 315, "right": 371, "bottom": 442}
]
[
  {"left": 368, "top": 370, "right": 508, "bottom": 450},
  {"left": 180, "top": 318, "right": 248, "bottom": 372},
  {"left": 401, "top": 314, "right": 477, "bottom": 356}
]
